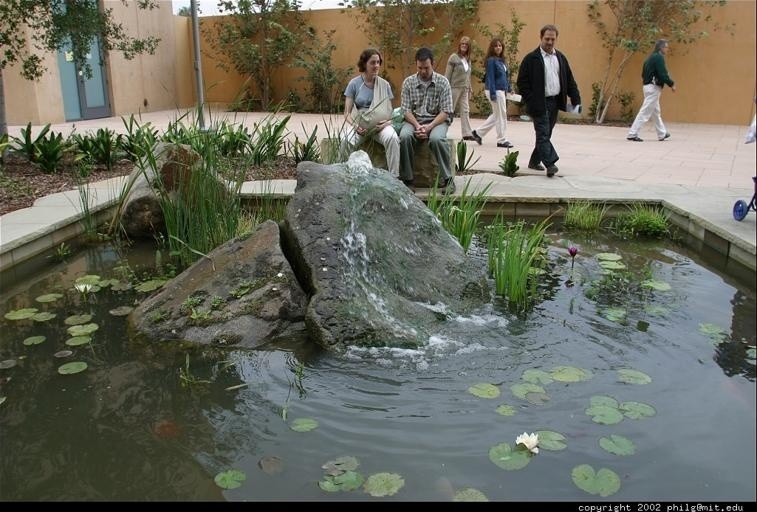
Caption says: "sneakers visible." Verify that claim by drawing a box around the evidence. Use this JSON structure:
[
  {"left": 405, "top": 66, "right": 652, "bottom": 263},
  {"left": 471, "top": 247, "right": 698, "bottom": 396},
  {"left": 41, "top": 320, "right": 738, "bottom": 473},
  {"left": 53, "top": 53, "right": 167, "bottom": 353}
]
[
  {"left": 659, "top": 133, "right": 670, "bottom": 141},
  {"left": 528, "top": 162, "right": 544, "bottom": 171},
  {"left": 442, "top": 178, "right": 456, "bottom": 195},
  {"left": 497, "top": 142, "right": 513, "bottom": 148},
  {"left": 463, "top": 130, "right": 482, "bottom": 145},
  {"left": 627, "top": 137, "right": 643, "bottom": 142},
  {"left": 547, "top": 164, "right": 558, "bottom": 178},
  {"left": 407, "top": 180, "right": 416, "bottom": 194}
]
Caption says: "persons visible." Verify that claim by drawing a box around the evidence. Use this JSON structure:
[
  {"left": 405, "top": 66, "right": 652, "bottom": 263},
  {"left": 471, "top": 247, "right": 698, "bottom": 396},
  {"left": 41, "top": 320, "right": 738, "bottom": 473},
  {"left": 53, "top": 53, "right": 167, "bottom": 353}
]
[
  {"left": 515, "top": 24, "right": 581, "bottom": 177},
  {"left": 471, "top": 37, "right": 514, "bottom": 148},
  {"left": 337, "top": 48, "right": 400, "bottom": 178},
  {"left": 627, "top": 39, "right": 676, "bottom": 141},
  {"left": 445, "top": 36, "right": 477, "bottom": 141},
  {"left": 399, "top": 47, "right": 456, "bottom": 196}
]
